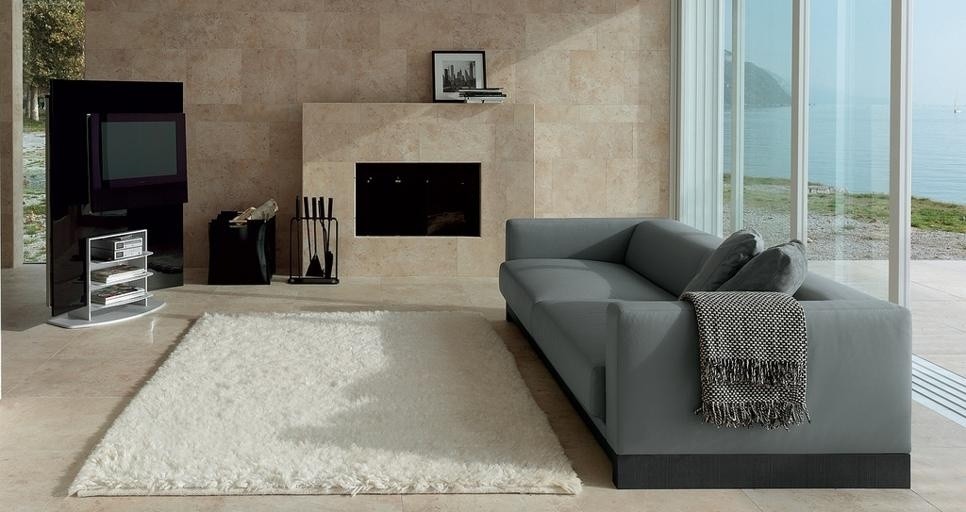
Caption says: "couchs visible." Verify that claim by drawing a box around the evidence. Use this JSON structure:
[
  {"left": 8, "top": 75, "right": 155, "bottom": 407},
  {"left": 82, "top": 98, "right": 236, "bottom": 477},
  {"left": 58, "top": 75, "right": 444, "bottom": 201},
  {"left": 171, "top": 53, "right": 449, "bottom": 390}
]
[{"left": 500, "top": 216, "right": 913, "bottom": 490}]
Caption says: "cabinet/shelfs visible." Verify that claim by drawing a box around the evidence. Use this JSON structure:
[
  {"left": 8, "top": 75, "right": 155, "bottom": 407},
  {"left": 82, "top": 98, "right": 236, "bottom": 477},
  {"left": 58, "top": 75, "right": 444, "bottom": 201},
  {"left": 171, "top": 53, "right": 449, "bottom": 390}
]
[{"left": 49, "top": 229, "right": 168, "bottom": 330}]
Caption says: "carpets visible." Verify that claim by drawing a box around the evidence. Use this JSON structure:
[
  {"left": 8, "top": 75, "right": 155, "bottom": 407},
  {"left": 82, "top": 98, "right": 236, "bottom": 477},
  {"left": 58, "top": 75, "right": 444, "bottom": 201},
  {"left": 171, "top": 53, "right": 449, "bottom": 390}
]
[{"left": 68, "top": 313, "right": 581, "bottom": 496}]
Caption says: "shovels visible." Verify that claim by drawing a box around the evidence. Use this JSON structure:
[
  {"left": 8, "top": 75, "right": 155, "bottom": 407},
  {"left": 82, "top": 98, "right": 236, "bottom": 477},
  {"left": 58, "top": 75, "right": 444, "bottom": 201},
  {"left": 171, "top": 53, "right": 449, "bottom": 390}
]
[{"left": 323, "top": 198, "right": 333, "bottom": 278}]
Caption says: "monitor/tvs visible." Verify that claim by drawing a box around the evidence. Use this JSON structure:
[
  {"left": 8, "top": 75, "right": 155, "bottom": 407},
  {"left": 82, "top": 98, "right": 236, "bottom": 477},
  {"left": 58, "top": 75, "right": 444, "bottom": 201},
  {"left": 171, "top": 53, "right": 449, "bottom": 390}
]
[{"left": 85, "top": 113, "right": 189, "bottom": 213}]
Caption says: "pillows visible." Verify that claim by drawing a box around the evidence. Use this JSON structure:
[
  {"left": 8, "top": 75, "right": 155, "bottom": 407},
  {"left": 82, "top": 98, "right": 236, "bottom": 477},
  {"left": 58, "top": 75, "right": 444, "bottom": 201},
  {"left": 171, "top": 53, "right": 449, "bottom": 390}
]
[
  {"left": 716, "top": 241, "right": 809, "bottom": 296},
  {"left": 676, "top": 229, "right": 763, "bottom": 296}
]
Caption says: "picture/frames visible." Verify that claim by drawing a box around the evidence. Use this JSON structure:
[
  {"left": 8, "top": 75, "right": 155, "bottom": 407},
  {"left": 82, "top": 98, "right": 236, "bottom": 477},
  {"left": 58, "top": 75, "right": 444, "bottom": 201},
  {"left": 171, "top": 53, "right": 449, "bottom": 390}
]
[{"left": 431, "top": 50, "right": 487, "bottom": 103}]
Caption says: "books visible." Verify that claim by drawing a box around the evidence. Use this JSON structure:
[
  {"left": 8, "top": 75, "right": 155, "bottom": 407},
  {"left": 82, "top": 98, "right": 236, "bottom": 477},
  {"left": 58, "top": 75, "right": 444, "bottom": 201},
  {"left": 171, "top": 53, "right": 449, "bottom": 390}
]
[
  {"left": 90, "top": 282, "right": 149, "bottom": 307},
  {"left": 459, "top": 88, "right": 508, "bottom": 104},
  {"left": 89, "top": 264, "right": 150, "bottom": 286},
  {"left": 111, "top": 237, "right": 143, "bottom": 260}
]
